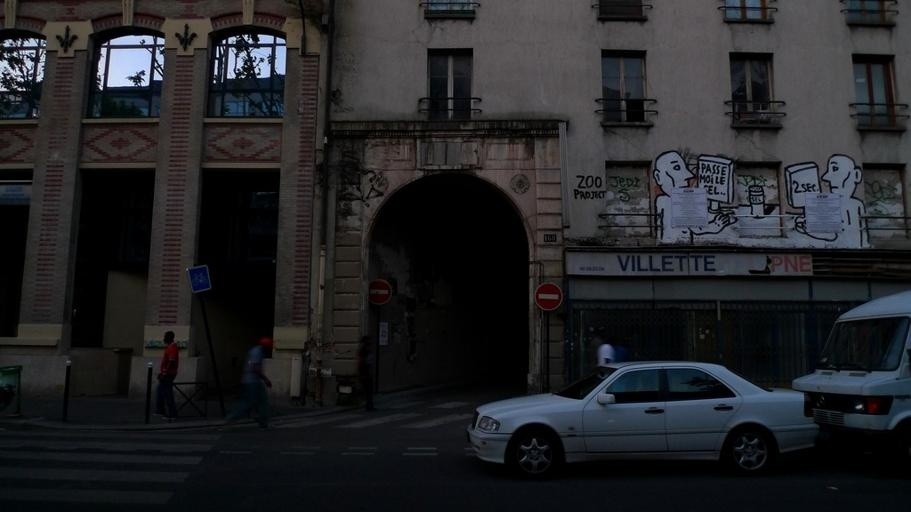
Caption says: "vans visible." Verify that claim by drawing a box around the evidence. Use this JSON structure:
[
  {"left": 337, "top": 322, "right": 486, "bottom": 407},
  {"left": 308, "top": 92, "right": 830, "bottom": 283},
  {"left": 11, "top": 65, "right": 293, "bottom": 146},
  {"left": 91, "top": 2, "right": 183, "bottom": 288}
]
[{"left": 791, "top": 290, "right": 911, "bottom": 471}]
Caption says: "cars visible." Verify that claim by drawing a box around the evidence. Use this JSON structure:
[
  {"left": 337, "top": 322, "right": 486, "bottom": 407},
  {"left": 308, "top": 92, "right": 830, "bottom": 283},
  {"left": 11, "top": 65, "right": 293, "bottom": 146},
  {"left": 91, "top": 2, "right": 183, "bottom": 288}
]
[{"left": 467, "top": 360, "right": 821, "bottom": 482}]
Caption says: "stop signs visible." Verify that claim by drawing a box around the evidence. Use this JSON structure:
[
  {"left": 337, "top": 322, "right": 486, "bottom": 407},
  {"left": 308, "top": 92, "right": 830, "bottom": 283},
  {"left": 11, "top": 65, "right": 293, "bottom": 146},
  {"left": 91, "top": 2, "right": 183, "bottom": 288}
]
[
  {"left": 369, "top": 279, "right": 392, "bottom": 306},
  {"left": 534, "top": 281, "right": 563, "bottom": 312}
]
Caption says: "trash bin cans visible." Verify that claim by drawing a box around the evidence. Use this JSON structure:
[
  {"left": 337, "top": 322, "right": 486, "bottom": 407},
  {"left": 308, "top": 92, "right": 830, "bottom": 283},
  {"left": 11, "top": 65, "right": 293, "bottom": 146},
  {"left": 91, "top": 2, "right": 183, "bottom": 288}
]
[{"left": 0, "top": 365, "right": 22, "bottom": 418}]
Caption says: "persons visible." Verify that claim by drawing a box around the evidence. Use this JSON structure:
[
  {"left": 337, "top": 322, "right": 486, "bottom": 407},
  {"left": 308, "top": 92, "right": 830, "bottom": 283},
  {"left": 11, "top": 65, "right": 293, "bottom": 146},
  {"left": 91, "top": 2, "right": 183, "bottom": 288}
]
[
  {"left": 355, "top": 335, "right": 380, "bottom": 411},
  {"left": 590, "top": 333, "right": 616, "bottom": 366},
  {"left": 222, "top": 336, "right": 277, "bottom": 429},
  {"left": 151, "top": 330, "right": 180, "bottom": 424}
]
